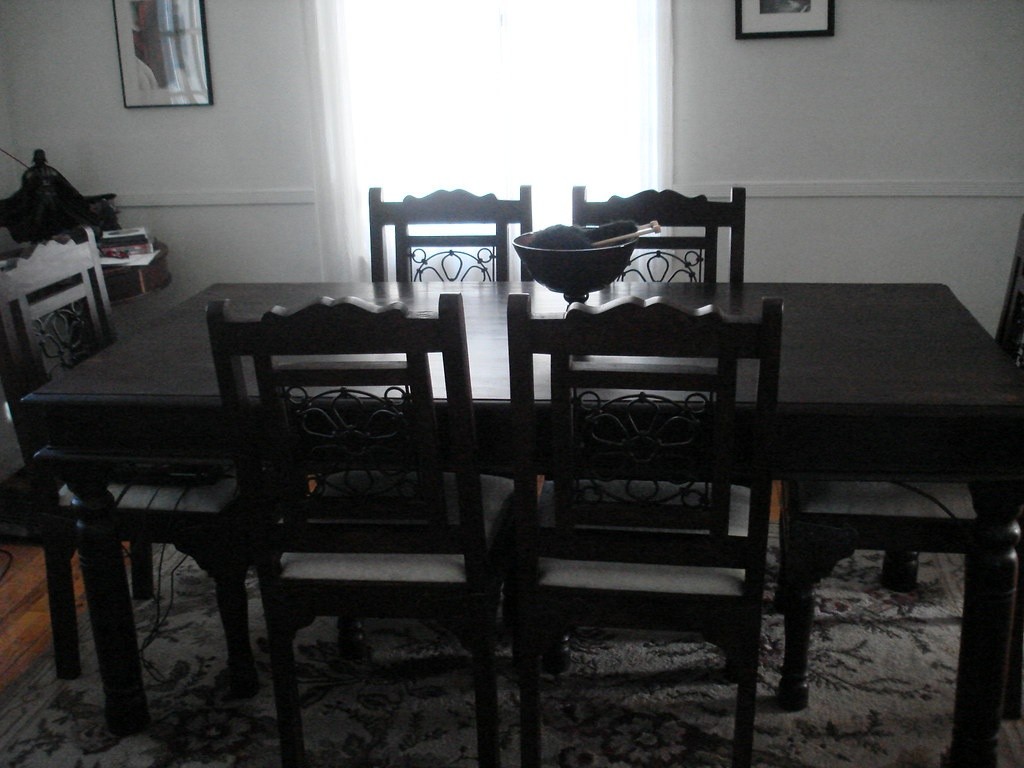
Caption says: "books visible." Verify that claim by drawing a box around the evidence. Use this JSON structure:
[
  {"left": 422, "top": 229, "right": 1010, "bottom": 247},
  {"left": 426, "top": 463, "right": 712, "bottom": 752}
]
[
  {"left": 100, "top": 227, "right": 149, "bottom": 247},
  {"left": 102, "top": 243, "right": 155, "bottom": 258}
]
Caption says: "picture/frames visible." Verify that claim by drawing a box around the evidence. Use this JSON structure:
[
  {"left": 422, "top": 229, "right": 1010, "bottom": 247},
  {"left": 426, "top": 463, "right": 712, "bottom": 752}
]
[{"left": 735, "top": 0, "right": 836, "bottom": 40}]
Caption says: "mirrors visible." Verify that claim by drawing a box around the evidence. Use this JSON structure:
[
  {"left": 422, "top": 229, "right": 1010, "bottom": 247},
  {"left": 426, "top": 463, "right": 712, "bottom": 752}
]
[{"left": 113, "top": 0, "right": 214, "bottom": 110}]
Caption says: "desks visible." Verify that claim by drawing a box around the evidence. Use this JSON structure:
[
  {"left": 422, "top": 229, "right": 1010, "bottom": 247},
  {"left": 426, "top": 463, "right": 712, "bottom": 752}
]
[
  {"left": 106, "top": 240, "right": 174, "bottom": 305},
  {"left": 21, "top": 280, "right": 1024, "bottom": 767}
]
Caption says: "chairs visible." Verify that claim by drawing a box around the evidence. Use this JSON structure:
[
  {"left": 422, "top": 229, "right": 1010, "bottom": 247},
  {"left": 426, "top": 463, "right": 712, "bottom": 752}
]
[
  {"left": 774, "top": 213, "right": 1024, "bottom": 722},
  {"left": 570, "top": 184, "right": 746, "bottom": 286},
  {"left": 509, "top": 292, "right": 785, "bottom": 768},
  {"left": 1, "top": 224, "right": 281, "bottom": 699},
  {"left": 369, "top": 185, "right": 534, "bottom": 284},
  {"left": 207, "top": 293, "right": 516, "bottom": 768}
]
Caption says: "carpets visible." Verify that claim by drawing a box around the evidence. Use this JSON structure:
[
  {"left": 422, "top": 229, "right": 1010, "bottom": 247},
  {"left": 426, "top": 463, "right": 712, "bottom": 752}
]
[{"left": 1, "top": 537, "right": 1024, "bottom": 768}]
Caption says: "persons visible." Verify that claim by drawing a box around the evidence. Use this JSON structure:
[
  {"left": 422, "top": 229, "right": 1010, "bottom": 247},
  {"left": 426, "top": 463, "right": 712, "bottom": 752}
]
[{"left": 22, "top": 149, "right": 70, "bottom": 243}]
